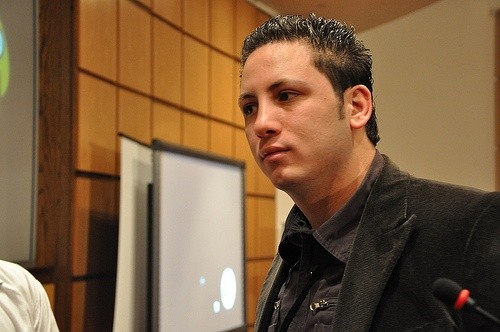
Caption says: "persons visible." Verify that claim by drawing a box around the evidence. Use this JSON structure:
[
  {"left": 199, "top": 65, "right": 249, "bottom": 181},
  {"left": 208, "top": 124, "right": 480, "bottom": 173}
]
[
  {"left": 0, "top": 259, "right": 58, "bottom": 332},
  {"left": 238, "top": 12, "right": 500, "bottom": 332}
]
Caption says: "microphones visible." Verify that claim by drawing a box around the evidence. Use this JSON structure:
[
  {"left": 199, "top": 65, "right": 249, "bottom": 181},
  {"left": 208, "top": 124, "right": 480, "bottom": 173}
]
[{"left": 431, "top": 278, "right": 500, "bottom": 327}]
